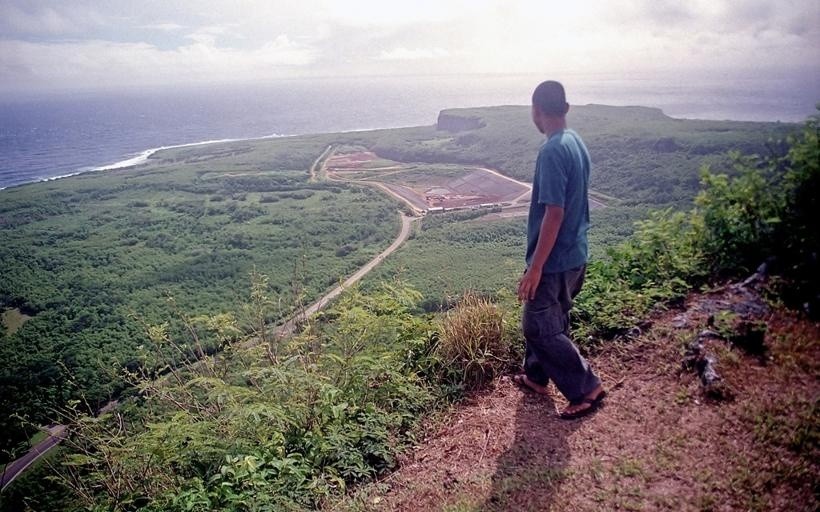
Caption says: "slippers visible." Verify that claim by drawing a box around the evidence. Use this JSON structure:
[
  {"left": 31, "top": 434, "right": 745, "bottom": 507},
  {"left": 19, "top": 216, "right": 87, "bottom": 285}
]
[
  {"left": 513, "top": 374, "right": 546, "bottom": 393},
  {"left": 562, "top": 391, "right": 605, "bottom": 419}
]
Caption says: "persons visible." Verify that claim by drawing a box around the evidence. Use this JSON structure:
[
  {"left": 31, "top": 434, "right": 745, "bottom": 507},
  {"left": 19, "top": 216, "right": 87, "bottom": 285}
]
[{"left": 511, "top": 80, "right": 610, "bottom": 420}]
